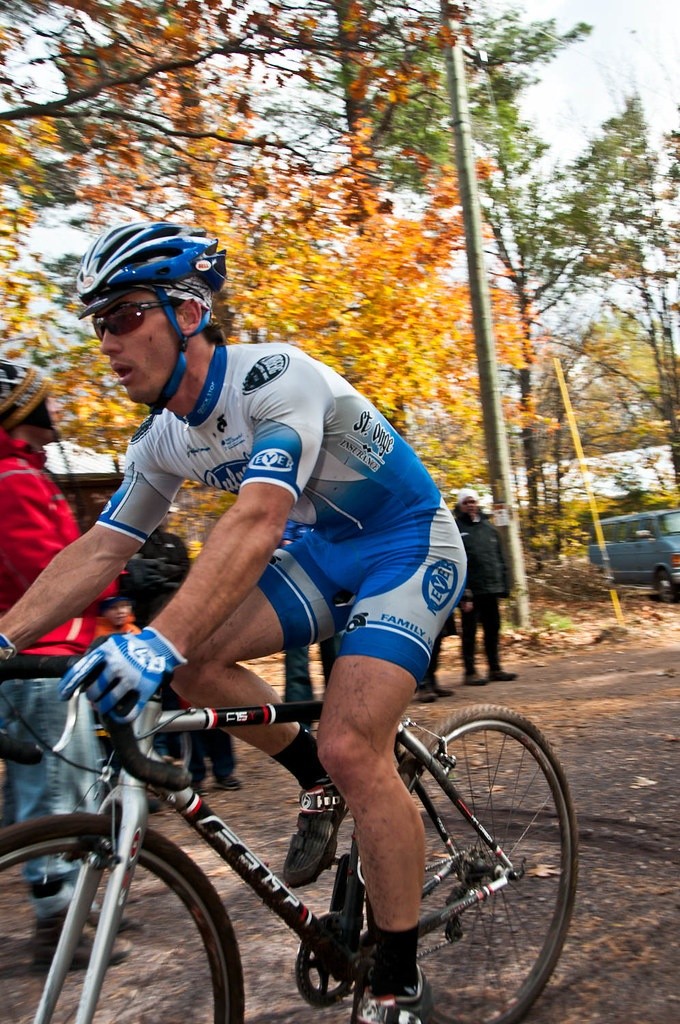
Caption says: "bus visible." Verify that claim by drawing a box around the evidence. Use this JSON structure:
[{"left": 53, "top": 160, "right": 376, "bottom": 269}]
[{"left": 581, "top": 509, "right": 680, "bottom": 601}]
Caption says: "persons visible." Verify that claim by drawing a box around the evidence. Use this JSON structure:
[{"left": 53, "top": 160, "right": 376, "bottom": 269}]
[
  {"left": 1, "top": 219, "right": 470, "bottom": 1024},
  {"left": 129, "top": 506, "right": 188, "bottom": 593},
  {"left": 415, "top": 615, "right": 457, "bottom": 700},
  {"left": 0, "top": 358, "right": 136, "bottom": 969},
  {"left": 90, "top": 595, "right": 143, "bottom": 644},
  {"left": 156, "top": 713, "right": 242, "bottom": 793},
  {"left": 283, "top": 634, "right": 341, "bottom": 724},
  {"left": 451, "top": 487, "right": 514, "bottom": 685}
]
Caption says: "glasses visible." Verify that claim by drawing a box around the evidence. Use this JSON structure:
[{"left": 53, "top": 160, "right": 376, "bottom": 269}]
[
  {"left": 462, "top": 500, "right": 475, "bottom": 505},
  {"left": 89, "top": 300, "right": 177, "bottom": 338}
]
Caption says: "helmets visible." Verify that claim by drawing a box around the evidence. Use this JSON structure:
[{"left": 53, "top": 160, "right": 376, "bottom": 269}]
[
  {"left": 0, "top": 360, "right": 56, "bottom": 432},
  {"left": 68, "top": 219, "right": 226, "bottom": 319}
]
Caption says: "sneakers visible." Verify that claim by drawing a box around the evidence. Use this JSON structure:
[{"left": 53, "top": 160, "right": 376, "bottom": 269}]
[
  {"left": 284, "top": 778, "right": 347, "bottom": 885},
  {"left": 351, "top": 967, "right": 433, "bottom": 1024}
]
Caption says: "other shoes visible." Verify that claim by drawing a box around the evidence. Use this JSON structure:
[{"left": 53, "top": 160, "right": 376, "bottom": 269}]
[
  {"left": 464, "top": 672, "right": 488, "bottom": 685},
  {"left": 418, "top": 686, "right": 436, "bottom": 702},
  {"left": 434, "top": 685, "right": 454, "bottom": 698},
  {"left": 489, "top": 668, "right": 516, "bottom": 681}
]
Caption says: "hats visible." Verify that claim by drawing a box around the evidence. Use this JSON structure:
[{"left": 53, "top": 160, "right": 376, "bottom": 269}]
[
  {"left": 102, "top": 586, "right": 131, "bottom": 615},
  {"left": 457, "top": 487, "right": 478, "bottom": 506}
]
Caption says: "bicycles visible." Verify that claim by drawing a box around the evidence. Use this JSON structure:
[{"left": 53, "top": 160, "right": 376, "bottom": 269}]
[{"left": 0, "top": 640, "right": 579, "bottom": 1023}]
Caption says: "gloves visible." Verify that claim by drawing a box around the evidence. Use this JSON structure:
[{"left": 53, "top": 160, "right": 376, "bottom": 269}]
[{"left": 55, "top": 624, "right": 188, "bottom": 721}]
